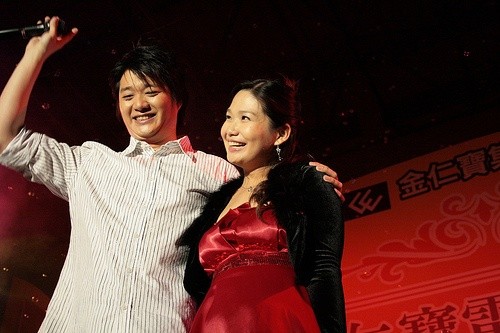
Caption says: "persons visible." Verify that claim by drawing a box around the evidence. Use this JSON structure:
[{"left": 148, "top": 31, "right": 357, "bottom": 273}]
[
  {"left": 0, "top": 13, "right": 347, "bottom": 332},
  {"left": 171, "top": 73, "right": 348, "bottom": 332}
]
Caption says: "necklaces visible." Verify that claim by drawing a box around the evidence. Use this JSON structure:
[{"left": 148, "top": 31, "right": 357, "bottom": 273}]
[{"left": 241, "top": 186, "right": 255, "bottom": 192}]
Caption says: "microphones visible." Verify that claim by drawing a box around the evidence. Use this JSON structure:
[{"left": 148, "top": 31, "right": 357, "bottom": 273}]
[{"left": 0, "top": 18, "right": 67, "bottom": 41}]
